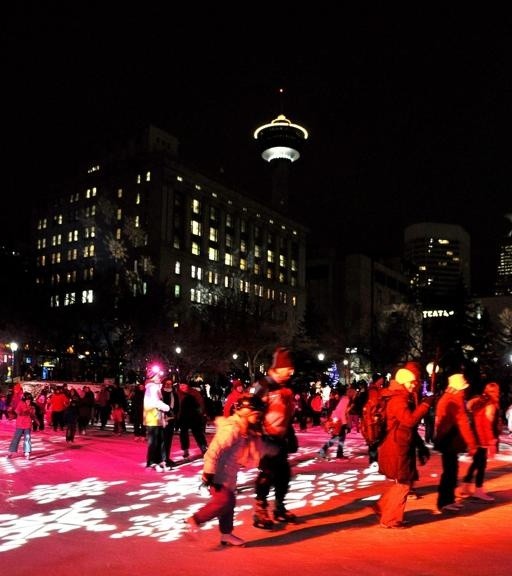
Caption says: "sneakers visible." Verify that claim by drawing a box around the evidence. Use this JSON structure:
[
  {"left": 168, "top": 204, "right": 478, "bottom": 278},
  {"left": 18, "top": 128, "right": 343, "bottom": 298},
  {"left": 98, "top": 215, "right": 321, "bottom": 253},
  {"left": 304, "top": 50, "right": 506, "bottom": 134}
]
[
  {"left": 252, "top": 509, "right": 274, "bottom": 526},
  {"left": 453, "top": 502, "right": 464, "bottom": 507},
  {"left": 187, "top": 517, "right": 201, "bottom": 541},
  {"left": 443, "top": 504, "right": 458, "bottom": 510},
  {"left": 221, "top": 533, "right": 244, "bottom": 546},
  {"left": 472, "top": 491, "right": 496, "bottom": 501},
  {"left": 273, "top": 509, "right": 297, "bottom": 521}
]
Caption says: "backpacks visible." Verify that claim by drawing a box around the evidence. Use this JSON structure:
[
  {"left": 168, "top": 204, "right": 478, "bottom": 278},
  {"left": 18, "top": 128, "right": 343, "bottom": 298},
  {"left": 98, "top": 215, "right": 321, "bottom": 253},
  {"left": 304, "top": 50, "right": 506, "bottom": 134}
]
[{"left": 360, "top": 388, "right": 411, "bottom": 447}]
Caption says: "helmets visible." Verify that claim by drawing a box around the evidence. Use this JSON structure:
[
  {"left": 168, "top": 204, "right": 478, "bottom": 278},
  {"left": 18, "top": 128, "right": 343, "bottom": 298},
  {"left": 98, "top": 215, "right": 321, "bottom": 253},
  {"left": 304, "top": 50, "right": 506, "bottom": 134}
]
[{"left": 202, "top": 473, "right": 215, "bottom": 487}]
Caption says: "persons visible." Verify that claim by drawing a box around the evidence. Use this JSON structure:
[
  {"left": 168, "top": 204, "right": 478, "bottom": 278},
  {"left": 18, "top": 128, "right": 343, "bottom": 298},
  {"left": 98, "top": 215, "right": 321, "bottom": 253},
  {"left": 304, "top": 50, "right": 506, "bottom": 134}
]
[{"left": 2, "top": 347, "right": 512, "bottom": 546}]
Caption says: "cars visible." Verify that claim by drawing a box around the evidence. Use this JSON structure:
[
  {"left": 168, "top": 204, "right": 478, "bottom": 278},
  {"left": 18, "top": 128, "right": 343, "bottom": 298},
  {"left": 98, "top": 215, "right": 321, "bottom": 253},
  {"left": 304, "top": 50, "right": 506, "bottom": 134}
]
[{"left": 24, "top": 366, "right": 48, "bottom": 380}]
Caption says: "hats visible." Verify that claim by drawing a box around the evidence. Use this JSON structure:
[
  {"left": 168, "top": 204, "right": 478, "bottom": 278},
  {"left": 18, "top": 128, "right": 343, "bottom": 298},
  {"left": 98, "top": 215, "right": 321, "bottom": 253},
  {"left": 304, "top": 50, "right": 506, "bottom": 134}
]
[
  {"left": 21, "top": 391, "right": 33, "bottom": 401},
  {"left": 447, "top": 373, "right": 470, "bottom": 391},
  {"left": 241, "top": 396, "right": 265, "bottom": 411},
  {"left": 147, "top": 370, "right": 159, "bottom": 380},
  {"left": 395, "top": 368, "right": 416, "bottom": 385},
  {"left": 271, "top": 347, "right": 295, "bottom": 369}
]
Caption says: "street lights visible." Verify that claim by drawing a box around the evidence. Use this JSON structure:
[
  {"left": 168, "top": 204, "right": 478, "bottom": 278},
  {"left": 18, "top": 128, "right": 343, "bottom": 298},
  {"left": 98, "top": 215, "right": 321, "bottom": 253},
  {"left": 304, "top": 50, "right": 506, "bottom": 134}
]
[{"left": 9, "top": 341, "right": 19, "bottom": 383}]
